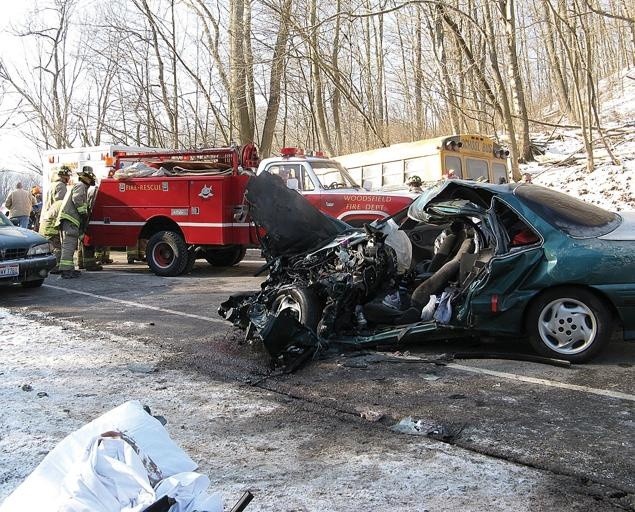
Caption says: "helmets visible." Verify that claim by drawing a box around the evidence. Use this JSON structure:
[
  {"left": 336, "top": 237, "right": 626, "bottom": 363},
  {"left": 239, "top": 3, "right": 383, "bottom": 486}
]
[
  {"left": 57, "top": 165, "right": 73, "bottom": 177},
  {"left": 76, "top": 166, "right": 96, "bottom": 186}
]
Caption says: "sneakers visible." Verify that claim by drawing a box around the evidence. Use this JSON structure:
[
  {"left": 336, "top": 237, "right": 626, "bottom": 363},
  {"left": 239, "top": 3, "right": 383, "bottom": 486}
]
[{"left": 49, "top": 256, "right": 114, "bottom": 279}]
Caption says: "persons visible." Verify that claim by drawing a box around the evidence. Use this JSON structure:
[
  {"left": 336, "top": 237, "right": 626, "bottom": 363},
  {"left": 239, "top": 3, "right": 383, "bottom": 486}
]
[
  {"left": 126, "top": 239, "right": 149, "bottom": 264},
  {"left": 405, "top": 168, "right": 535, "bottom": 198},
  {"left": 31, "top": 185, "right": 42, "bottom": 231},
  {"left": 4, "top": 180, "right": 38, "bottom": 229},
  {"left": 76, "top": 183, "right": 103, "bottom": 272},
  {"left": 37, "top": 165, "right": 72, "bottom": 224},
  {"left": 42, "top": 199, "right": 64, "bottom": 275},
  {"left": 94, "top": 245, "right": 114, "bottom": 265},
  {"left": 56, "top": 171, "right": 96, "bottom": 279}
]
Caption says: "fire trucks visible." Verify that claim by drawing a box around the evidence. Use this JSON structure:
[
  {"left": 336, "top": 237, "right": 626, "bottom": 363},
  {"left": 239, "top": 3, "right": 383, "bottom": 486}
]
[{"left": 42, "top": 143, "right": 422, "bottom": 277}]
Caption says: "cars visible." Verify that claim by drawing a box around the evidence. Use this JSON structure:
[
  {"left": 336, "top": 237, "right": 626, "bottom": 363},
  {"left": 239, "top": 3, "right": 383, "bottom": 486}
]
[
  {"left": 0, "top": 211, "right": 57, "bottom": 291},
  {"left": 216, "top": 169, "right": 635, "bottom": 364}
]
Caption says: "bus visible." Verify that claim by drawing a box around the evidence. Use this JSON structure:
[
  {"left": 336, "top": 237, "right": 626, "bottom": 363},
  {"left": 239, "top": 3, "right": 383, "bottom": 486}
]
[{"left": 291, "top": 134, "right": 511, "bottom": 197}]
